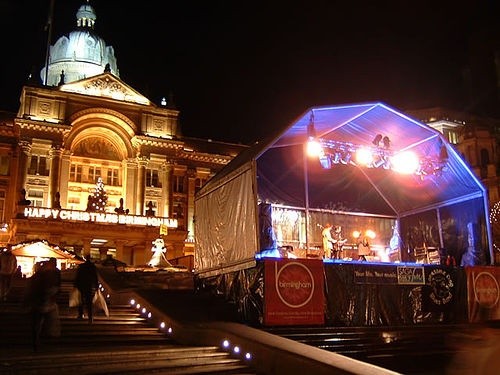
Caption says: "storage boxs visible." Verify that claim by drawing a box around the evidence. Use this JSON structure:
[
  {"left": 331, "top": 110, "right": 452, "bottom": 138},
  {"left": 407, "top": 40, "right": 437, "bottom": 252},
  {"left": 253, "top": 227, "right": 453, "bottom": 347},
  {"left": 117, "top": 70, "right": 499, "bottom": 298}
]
[{"left": 414, "top": 246, "right": 447, "bottom": 265}]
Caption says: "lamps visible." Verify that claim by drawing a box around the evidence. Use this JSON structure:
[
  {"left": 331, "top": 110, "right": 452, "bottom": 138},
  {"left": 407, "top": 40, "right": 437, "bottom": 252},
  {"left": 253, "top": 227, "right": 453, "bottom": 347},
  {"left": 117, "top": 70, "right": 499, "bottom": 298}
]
[{"left": 304, "top": 113, "right": 446, "bottom": 182}]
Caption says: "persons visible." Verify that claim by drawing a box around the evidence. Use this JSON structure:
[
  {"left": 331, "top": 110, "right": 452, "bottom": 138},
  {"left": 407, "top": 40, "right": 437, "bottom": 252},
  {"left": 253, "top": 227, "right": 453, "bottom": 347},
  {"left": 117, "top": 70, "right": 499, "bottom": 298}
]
[
  {"left": 321, "top": 223, "right": 337, "bottom": 260},
  {"left": 333, "top": 226, "right": 345, "bottom": 260},
  {"left": 74, "top": 253, "right": 99, "bottom": 324},
  {"left": 28, "top": 257, "right": 62, "bottom": 355}
]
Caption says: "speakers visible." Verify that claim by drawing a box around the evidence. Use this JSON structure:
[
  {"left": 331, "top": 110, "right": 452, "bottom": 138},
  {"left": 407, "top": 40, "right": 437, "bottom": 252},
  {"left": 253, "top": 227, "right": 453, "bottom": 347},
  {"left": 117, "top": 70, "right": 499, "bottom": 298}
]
[
  {"left": 259, "top": 203, "right": 273, "bottom": 248},
  {"left": 467, "top": 222, "right": 483, "bottom": 252}
]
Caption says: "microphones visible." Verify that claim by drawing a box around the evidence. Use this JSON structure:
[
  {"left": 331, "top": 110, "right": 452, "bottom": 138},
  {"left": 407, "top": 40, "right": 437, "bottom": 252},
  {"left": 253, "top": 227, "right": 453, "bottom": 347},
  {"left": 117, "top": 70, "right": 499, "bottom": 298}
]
[{"left": 317, "top": 224, "right": 322, "bottom": 227}]
